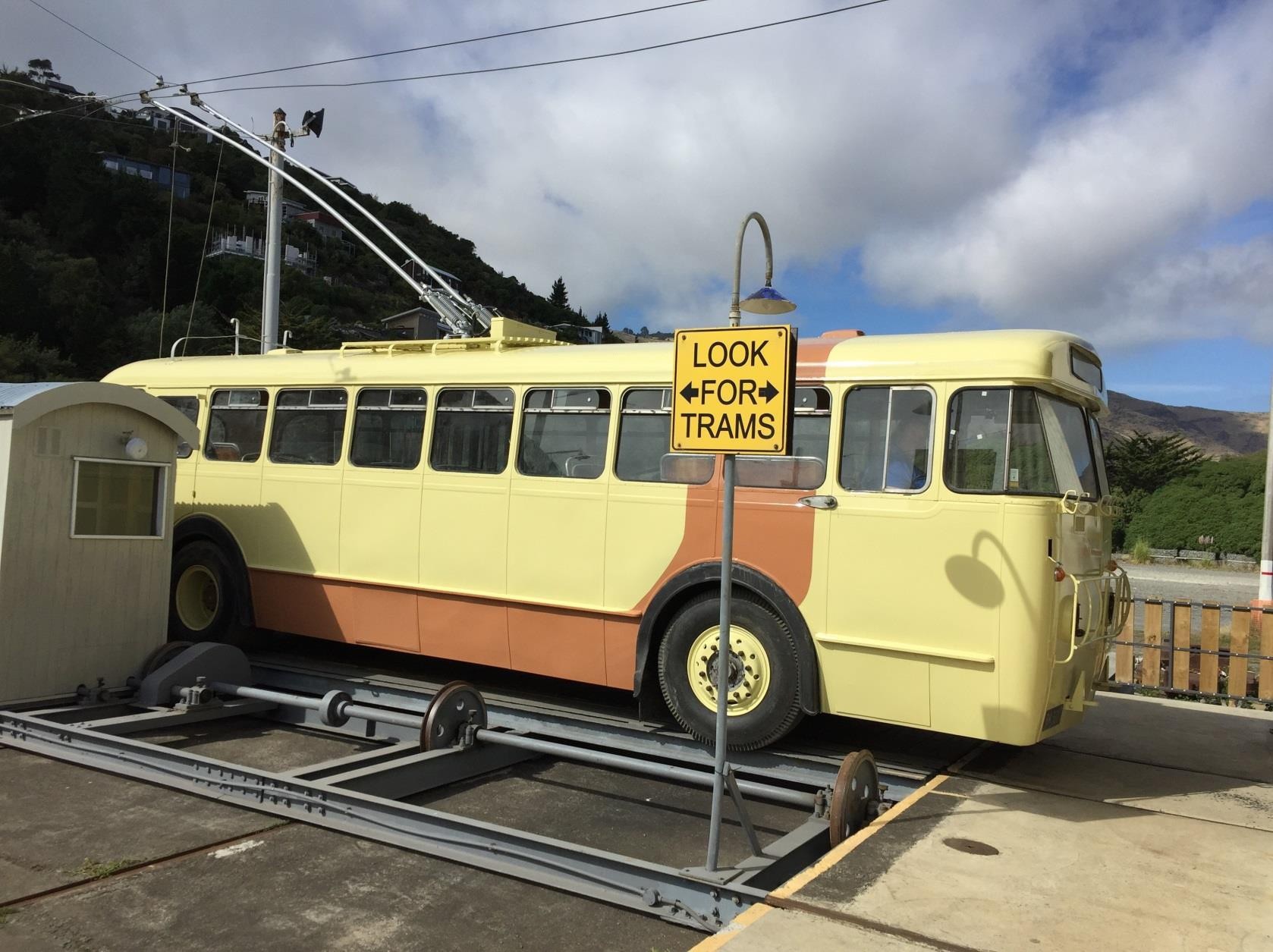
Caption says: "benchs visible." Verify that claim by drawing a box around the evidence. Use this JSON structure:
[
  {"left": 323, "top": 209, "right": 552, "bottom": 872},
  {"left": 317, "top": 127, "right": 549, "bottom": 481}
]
[{"left": 659, "top": 452, "right": 827, "bottom": 488}]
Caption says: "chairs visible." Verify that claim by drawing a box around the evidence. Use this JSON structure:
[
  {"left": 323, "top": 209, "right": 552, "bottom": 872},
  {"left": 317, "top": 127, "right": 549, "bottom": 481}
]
[
  {"left": 210, "top": 442, "right": 261, "bottom": 462},
  {"left": 565, "top": 453, "right": 603, "bottom": 479}
]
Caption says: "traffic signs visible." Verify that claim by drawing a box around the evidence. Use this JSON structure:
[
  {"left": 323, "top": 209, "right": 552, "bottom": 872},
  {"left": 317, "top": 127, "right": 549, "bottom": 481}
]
[{"left": 675, "top": 323, "right": 794, "bottom": 459}]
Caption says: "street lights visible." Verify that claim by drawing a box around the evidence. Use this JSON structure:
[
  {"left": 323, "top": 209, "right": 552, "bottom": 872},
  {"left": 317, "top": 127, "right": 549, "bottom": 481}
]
[
  {"left": 260, "top": 106, "right": 327, "bottom": 356},
  {"left": 709, "top": 213, "right": 799, "bottom": 883}
]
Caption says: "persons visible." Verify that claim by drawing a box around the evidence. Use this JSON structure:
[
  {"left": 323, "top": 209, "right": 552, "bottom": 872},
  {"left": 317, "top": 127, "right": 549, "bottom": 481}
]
[{"left": 875, "top": 420, "right": 927, "bottom": 491}]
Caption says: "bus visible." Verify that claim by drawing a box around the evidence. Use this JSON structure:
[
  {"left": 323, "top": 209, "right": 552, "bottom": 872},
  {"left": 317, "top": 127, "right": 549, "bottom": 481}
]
[{"left": 88, "top": 317, "right": 1137, "bottom": 755}]
